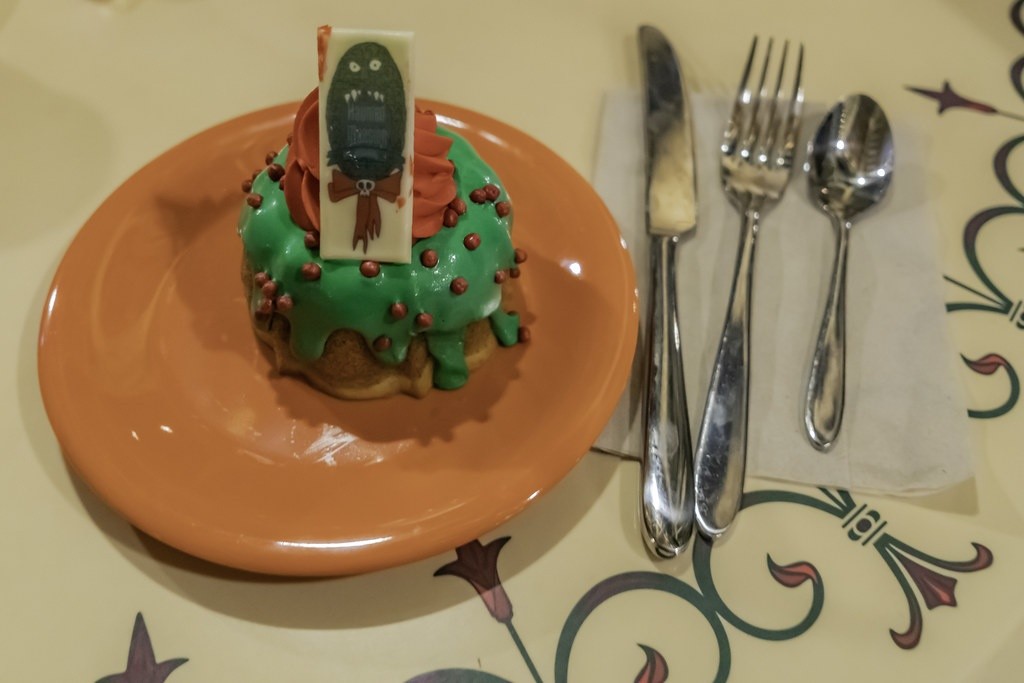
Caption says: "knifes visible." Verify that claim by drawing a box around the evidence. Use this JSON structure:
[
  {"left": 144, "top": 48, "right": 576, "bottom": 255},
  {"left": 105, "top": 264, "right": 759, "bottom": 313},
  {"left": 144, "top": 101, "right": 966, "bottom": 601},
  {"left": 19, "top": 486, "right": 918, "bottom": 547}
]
[{"left": 637, "top": 24, "right": 697, "bottom": 565}]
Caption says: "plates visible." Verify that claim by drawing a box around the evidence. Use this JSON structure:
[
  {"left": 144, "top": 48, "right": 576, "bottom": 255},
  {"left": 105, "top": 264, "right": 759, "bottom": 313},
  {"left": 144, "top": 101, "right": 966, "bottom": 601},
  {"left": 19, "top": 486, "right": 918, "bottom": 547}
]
[{"left": 41, "top": 110, "right": 637, "bottom": 581}]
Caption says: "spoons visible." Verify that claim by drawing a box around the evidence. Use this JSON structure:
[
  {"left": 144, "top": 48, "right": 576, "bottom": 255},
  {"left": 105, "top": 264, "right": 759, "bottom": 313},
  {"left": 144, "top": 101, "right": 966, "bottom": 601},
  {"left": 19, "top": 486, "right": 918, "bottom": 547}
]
[{"left": 804, "top": 91, "right": 897, "bottom": 453}]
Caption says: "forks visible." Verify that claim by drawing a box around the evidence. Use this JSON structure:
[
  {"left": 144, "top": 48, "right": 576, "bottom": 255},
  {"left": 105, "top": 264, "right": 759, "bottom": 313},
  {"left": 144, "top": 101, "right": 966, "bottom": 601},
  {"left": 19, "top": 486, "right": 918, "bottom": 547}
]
[{"left": 696, "top": 34, "right": 806, "bottom": 540}]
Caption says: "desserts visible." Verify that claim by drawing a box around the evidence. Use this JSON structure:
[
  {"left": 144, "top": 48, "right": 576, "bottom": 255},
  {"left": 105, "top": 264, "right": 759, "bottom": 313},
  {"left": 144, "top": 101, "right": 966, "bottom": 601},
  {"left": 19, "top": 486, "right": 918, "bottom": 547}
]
[{"left": 235, "top": 23, "right": 529, "bottom": 399}]
